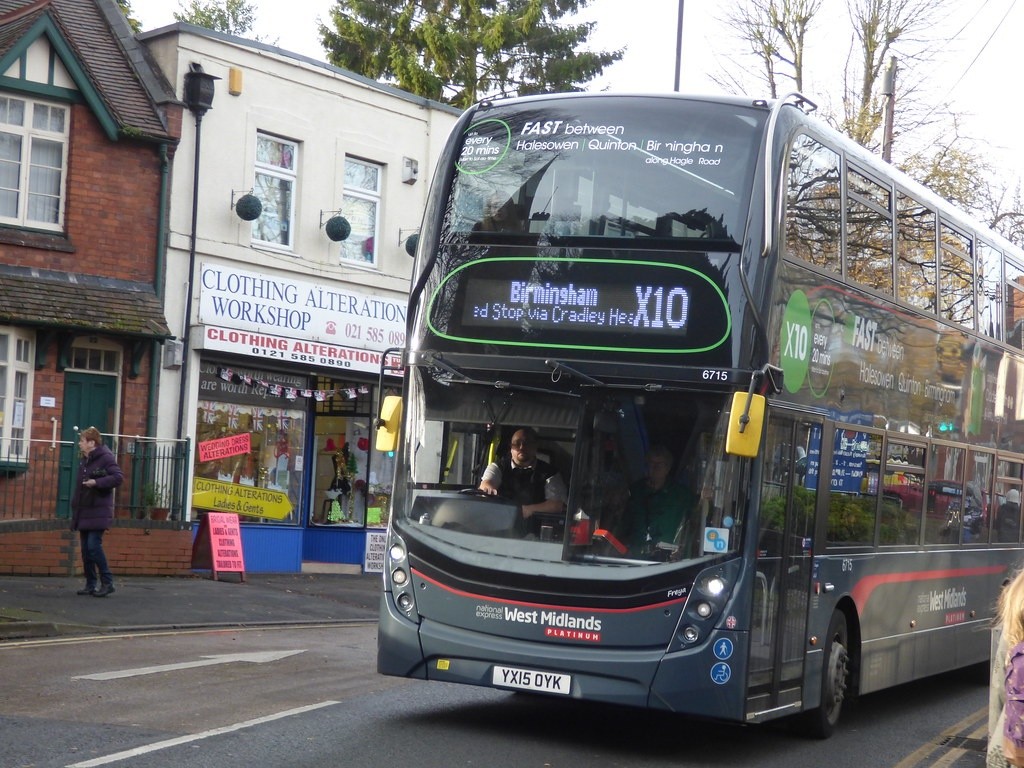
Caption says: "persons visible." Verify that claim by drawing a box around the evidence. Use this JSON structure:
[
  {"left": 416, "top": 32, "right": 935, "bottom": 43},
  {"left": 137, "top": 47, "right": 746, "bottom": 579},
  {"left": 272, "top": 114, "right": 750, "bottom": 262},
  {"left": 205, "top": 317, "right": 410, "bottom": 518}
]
[
  {"left": 480, "top": 428, "right": 565, "bottom": 533},
  {"left": 612, "top": 450, "right": 696, "bottom": 559},
  {"left": 945, "top": 486, "right": 981, "bottom": 543},
  {"left": 988, "top": 574, "right": 1024, "bottom": 768},
  {"left": 71, "top": 428, "right": 123, "bottom": 598},
  {"left": 996, "top": 489, "right": 1023, "bottom": 542},
  {"left": 471, "top": 191, "right": 520, "bottom": 232}
]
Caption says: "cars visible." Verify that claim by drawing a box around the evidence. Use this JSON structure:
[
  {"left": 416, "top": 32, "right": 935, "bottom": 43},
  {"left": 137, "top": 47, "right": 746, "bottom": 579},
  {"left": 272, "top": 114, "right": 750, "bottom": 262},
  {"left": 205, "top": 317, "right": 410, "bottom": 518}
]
[
  {"left": 861, "top": 458, "right": 919, "bottom": 494},
  {"left": 885, "top": 479, "right": 1006, "bottom": 527}
]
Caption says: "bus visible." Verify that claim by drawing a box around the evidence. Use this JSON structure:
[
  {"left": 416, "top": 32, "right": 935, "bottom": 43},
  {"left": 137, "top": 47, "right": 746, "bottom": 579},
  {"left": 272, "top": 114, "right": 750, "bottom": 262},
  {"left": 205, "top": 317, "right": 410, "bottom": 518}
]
[{"left": 378, "top": 92, "right": 1024, "bottom": 739}]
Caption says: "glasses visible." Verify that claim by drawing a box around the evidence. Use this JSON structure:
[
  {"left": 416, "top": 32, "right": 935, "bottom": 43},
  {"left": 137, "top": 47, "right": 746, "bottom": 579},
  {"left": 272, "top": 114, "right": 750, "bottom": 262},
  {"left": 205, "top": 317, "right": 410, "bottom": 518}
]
[{"left": 646, "top": 461, "right": 666, "bottom": 469}]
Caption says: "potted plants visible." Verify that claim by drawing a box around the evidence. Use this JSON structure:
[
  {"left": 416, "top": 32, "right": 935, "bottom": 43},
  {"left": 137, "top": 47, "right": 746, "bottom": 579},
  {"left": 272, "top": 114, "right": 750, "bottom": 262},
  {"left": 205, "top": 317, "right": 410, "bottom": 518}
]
[{"left": 143, "top": 482, "right": 169, "bottom": 520}]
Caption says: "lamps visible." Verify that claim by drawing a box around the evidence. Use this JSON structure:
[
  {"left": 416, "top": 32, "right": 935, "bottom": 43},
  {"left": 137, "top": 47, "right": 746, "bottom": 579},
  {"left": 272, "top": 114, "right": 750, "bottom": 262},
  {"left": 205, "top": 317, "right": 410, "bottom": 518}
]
[
  {"left": 398, "top": 226, "right": 420, "bottom": 257},
  {"left": 231, "top": 187, "right": 262, "bottom": 221},
  {"left": 319, "top": 208, "right": 351, "bottom": 241}
]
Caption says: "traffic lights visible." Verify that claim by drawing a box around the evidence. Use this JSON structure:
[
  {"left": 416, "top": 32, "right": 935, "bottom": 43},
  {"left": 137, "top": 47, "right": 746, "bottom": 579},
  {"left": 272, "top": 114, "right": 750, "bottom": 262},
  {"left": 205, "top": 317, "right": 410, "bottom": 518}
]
[{"left": 939, "top": 424, "right": 948, "bottom": 432}]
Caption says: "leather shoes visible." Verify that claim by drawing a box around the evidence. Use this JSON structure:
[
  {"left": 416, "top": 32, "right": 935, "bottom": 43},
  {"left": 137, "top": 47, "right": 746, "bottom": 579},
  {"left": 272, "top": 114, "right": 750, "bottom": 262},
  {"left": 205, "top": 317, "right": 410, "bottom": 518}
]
[
  {"left": 93, "top": 586, "right": 116, "bottom": 598},
  {"left": 77, "top": 589, "right": 96, "bottom": 596}
]
